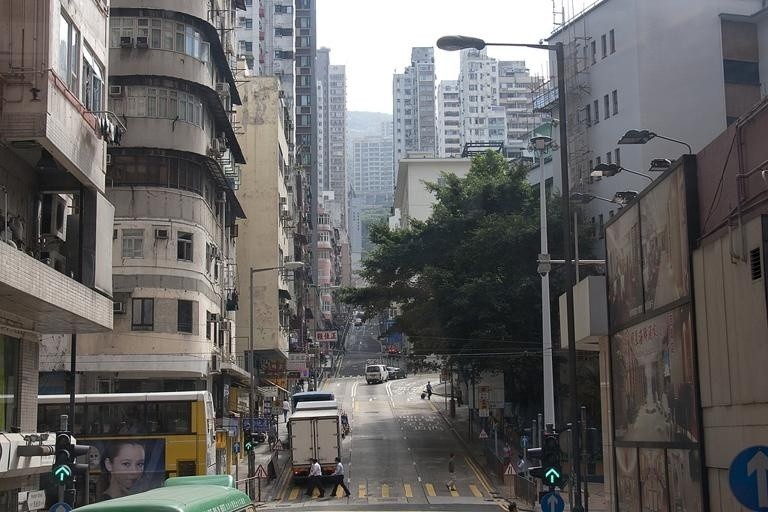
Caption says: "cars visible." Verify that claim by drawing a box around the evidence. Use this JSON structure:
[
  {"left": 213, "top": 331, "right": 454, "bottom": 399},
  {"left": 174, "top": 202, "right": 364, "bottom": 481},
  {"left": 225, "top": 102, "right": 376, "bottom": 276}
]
[
  {"left": 387, "top": 367, "right": 406, "bottom": 379},
  {"left": 355, "top": 318, "right": 362, "bottom": 326}
]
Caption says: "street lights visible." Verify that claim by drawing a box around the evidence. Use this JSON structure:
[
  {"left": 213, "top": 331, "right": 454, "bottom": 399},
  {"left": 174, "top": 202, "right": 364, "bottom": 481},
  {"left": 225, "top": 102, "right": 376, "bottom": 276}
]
[
  {"left": 314, "top": 286, "right": 340, "bottom": 391},
  {"left": 249, "top": 262, "right": 304, "bottom": 500}
]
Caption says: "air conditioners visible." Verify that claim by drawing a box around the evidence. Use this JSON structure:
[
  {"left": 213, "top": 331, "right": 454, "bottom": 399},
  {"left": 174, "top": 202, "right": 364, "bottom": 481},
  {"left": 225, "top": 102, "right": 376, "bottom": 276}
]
[
  {"left": 113, "top": 300, "right": 125, "bottom": 313},
  {"left": 109, "top": 84, "right": 122, "bottom": 96},
  {"left": 155, "top": 228, "right": 170, "bottom": 240},
  {"left": 118, "top": 33, "right": 149, "bottom": 51},
  {"left": 40, "top": 188, "right": 69, "bottom": 244}
]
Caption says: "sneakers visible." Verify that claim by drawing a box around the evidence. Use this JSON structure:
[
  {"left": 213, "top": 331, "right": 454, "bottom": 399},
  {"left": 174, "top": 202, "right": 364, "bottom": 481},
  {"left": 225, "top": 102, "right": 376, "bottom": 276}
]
[
  {"left": 446, "top": 485, "right": 456, "bottom": 491},
  {"left": 304, "top": 492, "right": 351, "bottom": 497}
]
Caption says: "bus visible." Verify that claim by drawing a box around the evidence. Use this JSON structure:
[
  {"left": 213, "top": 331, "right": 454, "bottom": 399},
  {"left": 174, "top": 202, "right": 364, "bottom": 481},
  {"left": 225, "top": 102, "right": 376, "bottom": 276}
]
[
  {"left": 37, "top": 390, "right": 216, "bottom": 503},
  {"left": 70, "top": 474, "right": 258, "bottom": 512}
]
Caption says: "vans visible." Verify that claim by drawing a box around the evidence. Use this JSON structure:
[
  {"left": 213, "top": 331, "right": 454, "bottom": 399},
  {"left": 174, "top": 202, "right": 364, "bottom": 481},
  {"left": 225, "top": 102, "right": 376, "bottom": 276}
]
[{"left": 365, "top": 365, "right": 389, "bottom": 384}]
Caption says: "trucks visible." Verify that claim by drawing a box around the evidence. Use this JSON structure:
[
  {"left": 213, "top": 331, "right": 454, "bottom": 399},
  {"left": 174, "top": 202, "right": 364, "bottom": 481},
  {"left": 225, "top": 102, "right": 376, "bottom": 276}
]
[{"left": 289, "top": 391, "right": 349, "bottom": 484}]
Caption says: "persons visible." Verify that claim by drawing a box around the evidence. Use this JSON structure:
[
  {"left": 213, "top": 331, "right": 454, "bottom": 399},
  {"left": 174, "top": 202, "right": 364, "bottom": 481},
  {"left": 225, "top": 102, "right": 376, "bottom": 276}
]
[
  {"left": 443, "top": 453, "right": 457, "bottom": 492},
  {"left": 455, "top": 386, "right": 463, "bottom": 407},
  {"left": 425, "top": 381, "right": 433, "bottom": 401},
  {"left": 469, "top": 397, "right": 531, "bottom": 477},
  {"left": 93, "top": 439, "right": 146, "bottom": 503},
  {"left": 507, "top": 501, "right": 518, "bottom": 512},
  {"left": 285, "top": 418, "right": 291, "bottom": 438},
  {"left": 326, "top": 457, "right": 351, "bottom": 498},
  {"left": 304, "top": 459, "right": 325, "bottom": 498},
  {"left": 281, "top": 398, "right": 289, "bottom": 424},
  {"left": 303, "top": 457, "right": 314, "bottom": 496}
]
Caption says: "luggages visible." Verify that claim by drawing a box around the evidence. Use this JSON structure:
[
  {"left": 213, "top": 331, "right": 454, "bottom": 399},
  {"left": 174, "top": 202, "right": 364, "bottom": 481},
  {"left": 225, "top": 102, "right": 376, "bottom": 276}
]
[{"left": 420, "top": 393, "right": 425, "bottom": 399}]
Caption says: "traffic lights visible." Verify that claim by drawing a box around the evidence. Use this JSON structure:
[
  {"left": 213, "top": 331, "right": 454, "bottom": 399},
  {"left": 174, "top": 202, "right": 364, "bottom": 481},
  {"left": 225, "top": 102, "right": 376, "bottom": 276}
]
[
  {"left": 244, "top": 430, "right": 253, "bottom": 451},
  {"left": 52, "top": 430, "right": 90, "bottom": 482},
  {"left": 541, "top": 430, "right": 562, "bottom": 486}
]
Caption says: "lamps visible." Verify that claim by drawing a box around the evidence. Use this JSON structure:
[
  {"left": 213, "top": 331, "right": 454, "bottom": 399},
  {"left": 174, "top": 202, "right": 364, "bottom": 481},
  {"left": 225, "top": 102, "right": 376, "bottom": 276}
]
[{"left": 568, "top": 124, "right": 691, "bottom": 210}]
